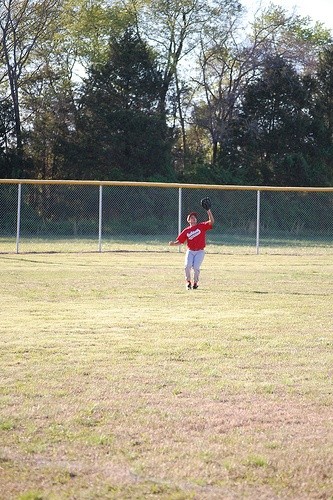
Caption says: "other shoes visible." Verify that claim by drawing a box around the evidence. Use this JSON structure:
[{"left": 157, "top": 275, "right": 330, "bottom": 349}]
[
  {"left": 187, "top": 283, "right": 191, "bottom": 290},
  {"left": 193, "top": 286, "right": 198, "bottom": 289}
]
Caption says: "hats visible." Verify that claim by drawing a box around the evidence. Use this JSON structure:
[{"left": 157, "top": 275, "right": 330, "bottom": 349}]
[{"left": 187, "top": 212, "right": 198, "bottom": 221}]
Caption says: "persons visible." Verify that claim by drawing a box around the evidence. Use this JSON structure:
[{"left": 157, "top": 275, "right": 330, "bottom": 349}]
[{"left": 169, "top": 197, "right": 214, "bottom": 289}]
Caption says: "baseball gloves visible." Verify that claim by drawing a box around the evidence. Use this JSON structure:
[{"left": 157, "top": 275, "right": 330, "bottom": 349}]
[{"left": 200, "top": 197, "right": 212, "bottom": 211}]
